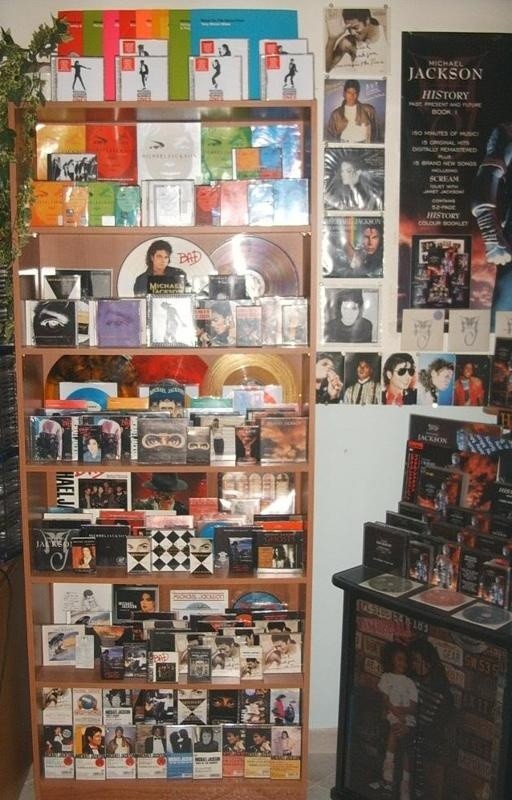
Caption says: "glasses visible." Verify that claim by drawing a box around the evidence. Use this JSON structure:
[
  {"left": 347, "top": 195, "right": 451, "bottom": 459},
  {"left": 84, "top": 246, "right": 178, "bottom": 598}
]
[{"left": 394, "top": 368, "right": 415, "bottom": 376}]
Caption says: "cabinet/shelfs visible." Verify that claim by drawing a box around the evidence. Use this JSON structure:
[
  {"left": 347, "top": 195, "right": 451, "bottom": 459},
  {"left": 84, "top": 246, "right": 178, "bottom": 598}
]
[{"left": 7, "top": 97, "right": 319, "bottom": 800}]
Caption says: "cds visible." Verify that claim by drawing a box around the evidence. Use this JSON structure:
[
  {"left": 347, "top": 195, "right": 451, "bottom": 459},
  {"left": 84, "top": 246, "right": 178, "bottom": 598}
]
[
  {"left": 463, "top": 606, "right": 510, "bottom": 625},
  {"left": 421, "top": 589, "right": 466, "bottom": 607},
  {"left": 370, "top": 575, "right": 414, "bottom": 592}
]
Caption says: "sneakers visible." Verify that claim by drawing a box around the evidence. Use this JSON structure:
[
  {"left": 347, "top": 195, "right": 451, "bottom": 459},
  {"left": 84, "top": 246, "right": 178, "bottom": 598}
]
[
  {"left": 399, "top": 780, "right": 410, "bottom": 800},
  {"left": 383, "top": 761, "right": 395, "bottom": 784}
]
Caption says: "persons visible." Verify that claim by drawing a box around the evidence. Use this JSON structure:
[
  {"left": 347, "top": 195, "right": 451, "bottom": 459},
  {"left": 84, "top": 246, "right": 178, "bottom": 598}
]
[
  {"left": 322, "top": 288, "right": 379, "bottom": 345},
  {"left": 84, "top": 439, "right": 101, "bottom": 463},
  {"left": 79, "top": 471, "right": 188, "bottom": 515},
  {"left": 283, "top": 58, "right": 298, "bottom": 88},
  {"left": 72, "top": 61, "right": 91, "bottom": 90},
  {"left": 43, "top": 687, "right": 296, "bottom": 759},
  {"left": 376, "top": 642, "right": 420, "bottom": 800},
  {"left": 205, "top": 418, "right": 235, "bottom": 455},
  {"left": 178, "top": 621, "right": 302, "bottom": 674},
  {"left": 155, "top": 296, "right": 308, "bottom": 347},
  {"left": 382, "top": 353, "right": 419, "bottom": 406},
  {"left": 314, "top": 351, "right": 343, "bottom": 404},
  {"left": 137, "top": 45, "right": 149, "bottom": 56},
  {"left": 323, "top": 149, "right": 385, "bottom": 213},
  {"left": 133, "top": 240, "right": 191, "bottom": 295},
  {"left": 126, "top": 538, "right": 150, "bottom": 552},
  {"left": 375, "top": 639, "right": 458, "bottom": 800},
  {"left": 325, "top": 79, "right": 387, "bottom": 145},
  {"left": 454, "top": 362, "right": 486, "bottom": 407},
  {"left": 79, "top": 546, "right": 95, "bottom": 570},
  {"left": 324, "top": 8, "right": 389, "bottom": 78},
  {"left": 211, "top": 60, "right": 221, "bottom": 89},
  {"left": 190, "top": 538, "right": 212, "bottom": 552},
  {"left": 470, "top": 118, "right": 511, "bottom": 331},
  {"left": 272, "top": 545, "right": 291, "bottom": 568},
  {"left": 138, "top": 592, "right": 154, "bottom": 611},
  {"left": 34, "top": 417, "right": 63, "bottom": 460},
  {"left": 31, "top": 123, "right": 251, "bottom": 226},
  {"left": 140, "top": 60, "right": 149, "bottom": 89},
  {"left": 219, "top": 44, "right": 231, "bottom": 56},
  {"left": 321, "top": 216, "right": 384, "bottom": 279},
  {"left": 96, "top": 301, "right": 140, "bottom": 349},
  {"left": 420, "top": 358, "right": 454, "bottom": 408},
  {"left": 408, "top": 451, "right": 512, "bottom": 612},
  {"left": 346, "top": 359, "right": 381, "bottom": 406}
]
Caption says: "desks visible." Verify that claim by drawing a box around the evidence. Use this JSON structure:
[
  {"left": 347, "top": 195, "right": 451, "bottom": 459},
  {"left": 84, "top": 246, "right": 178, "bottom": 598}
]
[{"left": 330, "top": 565, "right": 512, "bottom": 800}]
